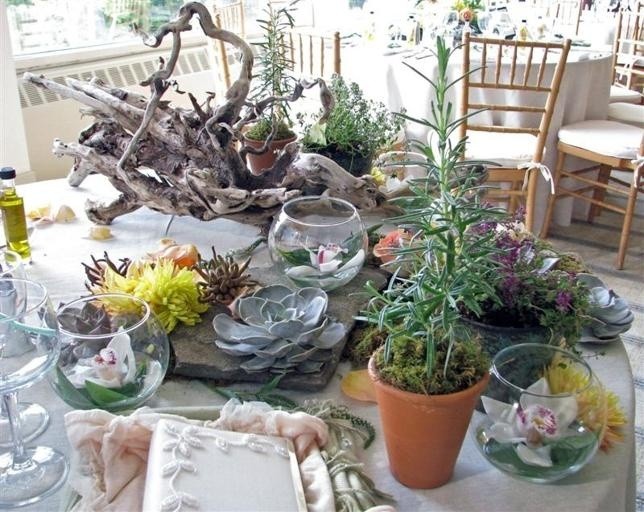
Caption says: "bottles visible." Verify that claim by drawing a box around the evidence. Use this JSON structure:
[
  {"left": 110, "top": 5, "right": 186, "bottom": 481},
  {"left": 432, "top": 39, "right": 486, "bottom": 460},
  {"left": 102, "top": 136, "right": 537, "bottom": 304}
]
[{"left": 0, "top": 166, "right": 32, "bottom": 259}]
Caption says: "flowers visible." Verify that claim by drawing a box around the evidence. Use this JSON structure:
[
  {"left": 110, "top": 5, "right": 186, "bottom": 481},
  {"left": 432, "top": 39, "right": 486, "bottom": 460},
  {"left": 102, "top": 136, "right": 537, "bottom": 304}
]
[
  {"left": 543, "top": 348, "right": 629, "bottom": 453},
  {"left": 210, "top": 285, "right": 347, "bottom": 377},
  {"left": 79, "top": 241, "right": 260, "bottom": 334},
  {"left": 450, "top": 0, "right": 486, "bottom": 22},
  {"left": 569, "top": 267, "right": 634, "bottom": 344},
  {"left": 464, "top": 202, "right": 605, "bottom": 351},
  {"left": 278, "top": 221, "right": 386, "bottom": 292},
  {"left": 480, "top": 376, "right": 599, "bottom": 481},
  {"left": 55, "top": 326, "right": 146, "bottom": 414}
]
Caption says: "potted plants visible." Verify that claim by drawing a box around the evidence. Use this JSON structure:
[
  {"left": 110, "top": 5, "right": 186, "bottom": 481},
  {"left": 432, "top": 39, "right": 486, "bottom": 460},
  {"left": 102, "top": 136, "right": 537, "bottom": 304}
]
[
  {"left": 242, "top": 0, "right": 307, "bottom": 175},
  {"left": 305, "top": 75, "right": 408, "bottom": 178},
  {"left": 349, "top": 35, "right": 520, "bottom": 491}
]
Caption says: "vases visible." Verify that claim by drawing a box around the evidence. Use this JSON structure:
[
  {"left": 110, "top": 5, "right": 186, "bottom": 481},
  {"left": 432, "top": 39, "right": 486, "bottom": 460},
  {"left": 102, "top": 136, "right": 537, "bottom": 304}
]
[
  {"left": 461, "top": 310, "right": 533, "bottom": 353},
  {"left": 471, "top": 341, "right": 608, "bottom": 485},
  {"left": 44, "top": 294, "right": 171, "bottom": 414},
  {"left": 267, "top": 197, "right": 370, "bottom": 293},
  {"left": 462, "top": 22, "right": 472, "bottom": 44}
]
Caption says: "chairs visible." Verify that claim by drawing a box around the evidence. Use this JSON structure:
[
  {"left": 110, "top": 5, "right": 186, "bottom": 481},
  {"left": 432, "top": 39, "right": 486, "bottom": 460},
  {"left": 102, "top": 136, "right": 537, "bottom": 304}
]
[
  {"left": 287, "top": 30, "right": 341, "bottom": 105},
  {"left": 459, "top": 30, "right": 572, "bottom": 235},
  {"left": 521, "top": 0, "right": 644, "bottom": 271},
  {"left": 212, "top": 0, "right": 244, "bottom": 93}
]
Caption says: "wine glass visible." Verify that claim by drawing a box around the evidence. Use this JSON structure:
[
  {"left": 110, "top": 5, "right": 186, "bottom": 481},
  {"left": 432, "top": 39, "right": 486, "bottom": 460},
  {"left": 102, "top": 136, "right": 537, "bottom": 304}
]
[
  {"left": 1, "top": 250, "right": 50, "bottom": 445},
  {"left": 1, "top": 285, "right": 69, "bottom": 507}
]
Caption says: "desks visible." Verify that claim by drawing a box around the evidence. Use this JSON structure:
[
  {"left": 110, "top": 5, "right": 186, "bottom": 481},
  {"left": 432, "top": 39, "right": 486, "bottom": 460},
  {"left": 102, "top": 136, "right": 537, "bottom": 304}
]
[{"left": 0, "top": 166, "right": 637, "bottom": 512}]
